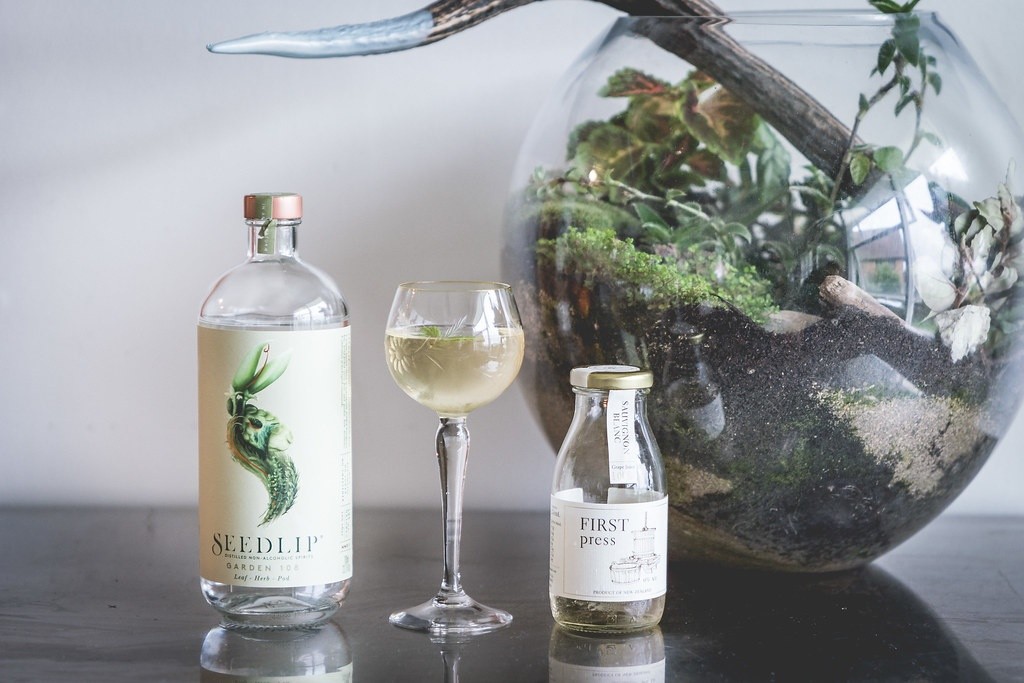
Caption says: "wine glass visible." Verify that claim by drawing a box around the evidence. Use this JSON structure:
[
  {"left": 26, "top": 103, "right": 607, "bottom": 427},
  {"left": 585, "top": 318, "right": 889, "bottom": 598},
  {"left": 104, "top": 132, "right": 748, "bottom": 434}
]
[
  {"left": 425, "top": 625, "right": 510, "bottom": 683},
  {"left": 384, "top": 281, "right": 525, "bottom": 634}
]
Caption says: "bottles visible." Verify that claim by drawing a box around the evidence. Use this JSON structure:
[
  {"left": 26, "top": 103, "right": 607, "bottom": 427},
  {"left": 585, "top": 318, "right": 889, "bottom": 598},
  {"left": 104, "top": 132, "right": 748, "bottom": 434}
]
[
  {"left": 196, "top": 192, "right": 353, "bottom": 630},
  {"left": 200, "top": 619, "right": 354, "bottom": 683},
  {"left": 549, "top": 623, "right": 666, "bottom": 683},
  {"left": 548, "top": 365, "right": 668, "bottom": 635}
]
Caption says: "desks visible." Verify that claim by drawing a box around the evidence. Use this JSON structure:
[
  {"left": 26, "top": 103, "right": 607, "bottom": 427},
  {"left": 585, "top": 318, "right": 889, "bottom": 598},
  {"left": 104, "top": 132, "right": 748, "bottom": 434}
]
[{"left": 0, "top": 507, "right": 1024, "bottom": 683}]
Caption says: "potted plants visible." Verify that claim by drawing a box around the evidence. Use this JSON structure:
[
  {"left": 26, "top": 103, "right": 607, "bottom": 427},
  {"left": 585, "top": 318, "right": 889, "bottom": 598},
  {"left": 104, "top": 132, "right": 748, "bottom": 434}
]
[{"left": 204, "top": 0, "right": 1024, "bottom": 576}]
